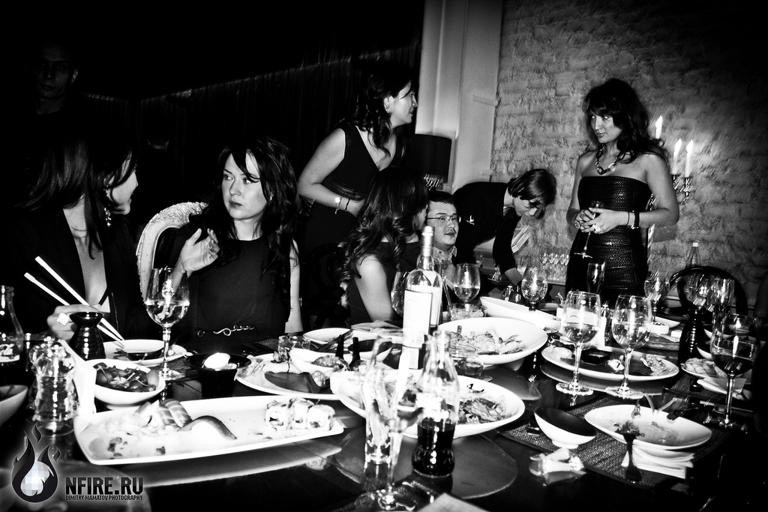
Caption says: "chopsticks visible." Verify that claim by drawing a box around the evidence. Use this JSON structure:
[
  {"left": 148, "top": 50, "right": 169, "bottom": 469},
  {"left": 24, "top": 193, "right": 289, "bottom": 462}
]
[{"left": 24, "top": 255, "right": 130, "bottom": 348}]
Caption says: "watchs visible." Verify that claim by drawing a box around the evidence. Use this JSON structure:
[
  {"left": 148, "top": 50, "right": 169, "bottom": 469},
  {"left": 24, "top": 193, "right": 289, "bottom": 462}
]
[{"left": 631, "top": 209, "right": 641, "bottom": 230}]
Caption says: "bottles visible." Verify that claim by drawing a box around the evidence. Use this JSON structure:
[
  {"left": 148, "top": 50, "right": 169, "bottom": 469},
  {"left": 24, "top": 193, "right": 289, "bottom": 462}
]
[
  {"left": 30, "top": 337, "right": 79, "bottom": 439},
  {"left": 411, "top": 330, "right": 460, "bottom": 479},
  {"left": 0, "top": 286, "right": 28, "bottom": 385}
]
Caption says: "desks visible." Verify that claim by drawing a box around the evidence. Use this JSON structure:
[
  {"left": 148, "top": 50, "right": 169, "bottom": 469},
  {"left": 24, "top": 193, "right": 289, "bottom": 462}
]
[{"left": 1, "top": 292, "right": 762, "bottom": 511}]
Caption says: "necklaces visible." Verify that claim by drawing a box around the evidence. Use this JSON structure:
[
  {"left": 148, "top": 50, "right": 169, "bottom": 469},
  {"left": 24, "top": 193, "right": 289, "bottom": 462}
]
[{"left": 596, "top": 157, "right": 618, "bottom": 175}]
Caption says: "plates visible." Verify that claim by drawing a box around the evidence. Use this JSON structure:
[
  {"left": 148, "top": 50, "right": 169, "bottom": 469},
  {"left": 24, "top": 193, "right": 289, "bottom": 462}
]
[
  {"left": 72, "top": 296, "right": 766, "bottom": 467},
  {"left": 332, "top": 424, "right": 519, "bottom": 500},
  {"left": 110, "top": 439, "right": 342, "bottom": 489}
]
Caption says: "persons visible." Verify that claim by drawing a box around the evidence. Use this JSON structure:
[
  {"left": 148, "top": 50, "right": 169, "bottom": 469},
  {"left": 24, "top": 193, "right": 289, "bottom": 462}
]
[
  {"left": 6, "top": 126, "right": 224, "bottom": 343},
  {"left": 174, "top": 133, "right": 306, "bottom": 338},
  {"left": 564, "top": 74, "right": 679, "bottom": 307},
  {"left": 450, "top": 167, "right": 556, "bottom": 294},
  {"left": 337, "top": 167, "right": 430, "bottom": 323},
  {"left": 298, "top": 70, "right": 419, "bottom": 326},
  {"left": 424, "top": 189, "right": 477, "bottom": 312}
]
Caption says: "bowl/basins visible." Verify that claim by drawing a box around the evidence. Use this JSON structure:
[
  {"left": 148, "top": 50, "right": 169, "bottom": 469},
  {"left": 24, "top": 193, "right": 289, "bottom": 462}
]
[{"left": 0, "top": 385, "right": 29, "bottom": 428}]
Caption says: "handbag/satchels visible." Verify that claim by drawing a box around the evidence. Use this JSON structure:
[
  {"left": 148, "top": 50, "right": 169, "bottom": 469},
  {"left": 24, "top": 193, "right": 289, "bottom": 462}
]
[{"left": 677, "top": 268, "right": 748, "bottom": 323}]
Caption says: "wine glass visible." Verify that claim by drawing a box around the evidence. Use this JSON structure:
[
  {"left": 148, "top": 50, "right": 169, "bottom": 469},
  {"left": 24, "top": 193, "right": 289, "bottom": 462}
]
[{"left": 144, "top": 201, "right": 761, "bottom": 434}]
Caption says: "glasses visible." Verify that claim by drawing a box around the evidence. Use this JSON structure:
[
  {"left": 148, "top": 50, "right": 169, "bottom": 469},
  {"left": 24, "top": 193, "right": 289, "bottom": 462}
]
[{"left": 426, "top": 216, "right": 462, "bottom": 224}]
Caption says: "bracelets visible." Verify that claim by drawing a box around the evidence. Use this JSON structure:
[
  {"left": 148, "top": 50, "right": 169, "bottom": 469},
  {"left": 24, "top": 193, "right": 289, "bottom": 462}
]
[
  {"left": 334, "top": 196, "right": 342, "bottom": 214},
  {"left": 625, "top": 211, "right": 630, "bottom": 226},
  {"left": 344, "top": 198, "right": 350, "bottom": 213}
]
